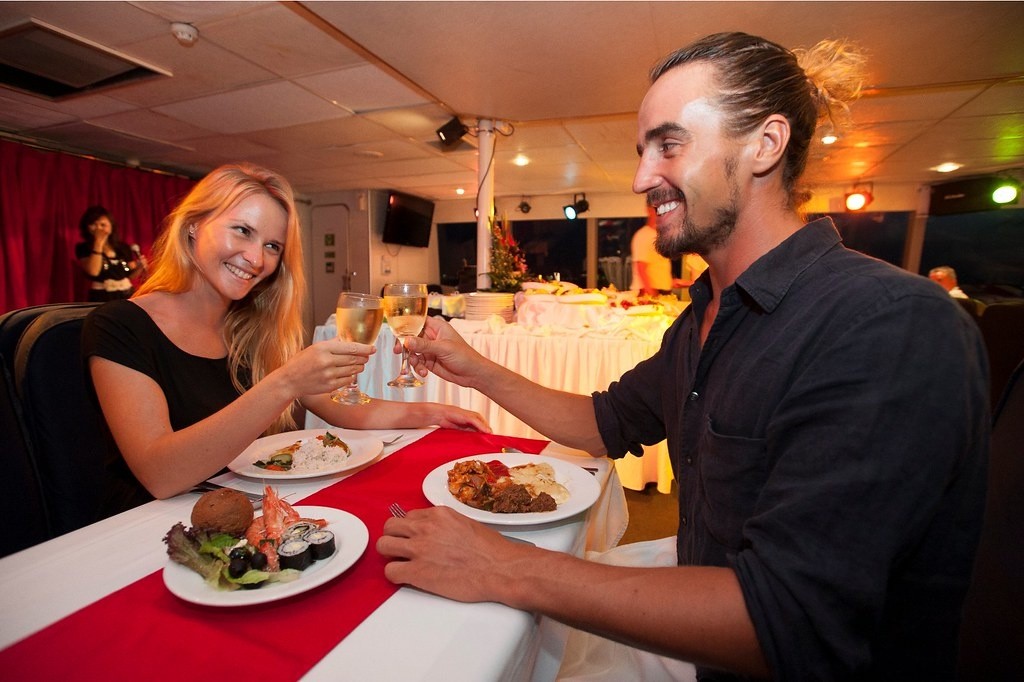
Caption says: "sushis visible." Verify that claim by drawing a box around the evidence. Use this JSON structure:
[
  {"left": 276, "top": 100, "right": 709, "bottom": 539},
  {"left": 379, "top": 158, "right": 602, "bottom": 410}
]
[{"left": 276, "top": 521, "right": 335, "bottom": 571}]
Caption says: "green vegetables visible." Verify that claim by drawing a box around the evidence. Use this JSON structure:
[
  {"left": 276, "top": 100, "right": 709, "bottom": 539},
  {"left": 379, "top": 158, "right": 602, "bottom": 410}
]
[
  {"left": 253, "top": 458, "right": 293, "bottom": 470},
  {"left": 162, "top": 522, "right": 302, "bottom": 590}
]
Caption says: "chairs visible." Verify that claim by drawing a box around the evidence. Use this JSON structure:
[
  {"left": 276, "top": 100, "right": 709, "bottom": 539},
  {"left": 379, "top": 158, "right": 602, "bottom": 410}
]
[
  {"left": 955, "top": 357, "right": 1024, "bottom": 682},
  {"left": 0, "top": 302, "right": 105, "bottom": 556}
]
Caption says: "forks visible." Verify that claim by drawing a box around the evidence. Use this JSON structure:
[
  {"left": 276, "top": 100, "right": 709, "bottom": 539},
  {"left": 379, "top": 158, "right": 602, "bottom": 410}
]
[
  {"left": 383, "top": 434, "right": 404, "bottom": 446},
  {"left": 389, "top": 503, "right": 406, "bottom": 518}
]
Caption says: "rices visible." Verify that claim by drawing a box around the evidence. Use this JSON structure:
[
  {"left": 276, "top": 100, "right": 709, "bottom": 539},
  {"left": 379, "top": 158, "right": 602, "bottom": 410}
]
[{"left": 290, "top": 438, "right": 350, "bottom": 473}]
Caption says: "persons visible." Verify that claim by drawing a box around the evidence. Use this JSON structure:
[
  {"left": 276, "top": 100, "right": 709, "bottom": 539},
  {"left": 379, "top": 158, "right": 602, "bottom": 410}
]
[
  {"left": 377, "top": 32, "right": 991, "bottom": 681},
  {"left": 686, "top": 254, "right": 708, "bottom": 281},
  {"left": 927, "top": 267, "right": 969, "bottom": 299},
  {"left": 81, "top": 164, "right": 492, "bottom": 542},
  {"left": 77, "top": 206, "right": 147, "bottom": 300},
  {"left": 630, "top": 202, "right": 671, "bottom": 296}
]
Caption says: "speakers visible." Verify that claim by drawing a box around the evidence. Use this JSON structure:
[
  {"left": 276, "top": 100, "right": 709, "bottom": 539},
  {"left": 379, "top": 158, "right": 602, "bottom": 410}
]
[{"left": 436, "top": 116, "right": 467, "bottom": 145}]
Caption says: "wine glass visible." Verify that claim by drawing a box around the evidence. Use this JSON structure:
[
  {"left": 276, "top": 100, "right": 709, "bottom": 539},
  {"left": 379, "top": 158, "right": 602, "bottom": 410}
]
[
  {"left": 328, "top": 291, "right": 383, "bottom": 407},
  {"left": 383, "top": 283, "right": 429, "bottom": 389}
]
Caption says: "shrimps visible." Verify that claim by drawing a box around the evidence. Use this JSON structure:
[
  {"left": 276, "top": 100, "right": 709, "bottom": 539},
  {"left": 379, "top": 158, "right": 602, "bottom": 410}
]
[{"left": 247, "top": 484, "right": 328, "bottom": 572}]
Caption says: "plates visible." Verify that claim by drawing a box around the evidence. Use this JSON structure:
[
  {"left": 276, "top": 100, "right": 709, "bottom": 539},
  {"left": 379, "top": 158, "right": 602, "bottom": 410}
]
[
  {"left": 162, "top": 506, "right": 369, "bottom": 608},
  {"left": 227, "top": 427, "right": 383, "bottom": 481},
  {"left": 465, "top": 291, "right": 515, "bottom": 324},
  {"left": 422, "top": 452, "right": 603, "bottom": 526}
]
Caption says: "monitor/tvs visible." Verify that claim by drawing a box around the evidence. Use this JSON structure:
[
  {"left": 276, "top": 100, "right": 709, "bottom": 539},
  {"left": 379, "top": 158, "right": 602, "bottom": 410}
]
[{"left": 382, "top": 190, "right": 435, "bottom": 248}]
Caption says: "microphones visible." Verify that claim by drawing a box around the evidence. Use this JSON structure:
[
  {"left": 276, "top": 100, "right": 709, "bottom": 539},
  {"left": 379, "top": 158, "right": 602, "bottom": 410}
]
[{"left": 130, "top": 244, "right": 142, "bottom": 260}]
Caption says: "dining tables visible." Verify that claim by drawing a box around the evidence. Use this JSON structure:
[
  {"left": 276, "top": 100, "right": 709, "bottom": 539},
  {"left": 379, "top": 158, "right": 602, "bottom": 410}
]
[
  {"left": 0, "top": 427, "right": 632, "bottom": 682},
  {"left": 303, "top": 318, "right": 676, "bottom": 496}
]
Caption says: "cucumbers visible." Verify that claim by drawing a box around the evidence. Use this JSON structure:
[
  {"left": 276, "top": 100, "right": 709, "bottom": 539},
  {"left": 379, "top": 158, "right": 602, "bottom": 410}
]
[{"left": 271, "top": 454, "right": 292, "bottom": 463}]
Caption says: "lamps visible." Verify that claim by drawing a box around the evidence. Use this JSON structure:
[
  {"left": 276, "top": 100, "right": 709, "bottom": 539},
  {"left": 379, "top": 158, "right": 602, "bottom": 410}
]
[
  {"left": 435, "top": 116, "right": 478, "bottom": 148},
  {"left": 473, "top": 196, "right": 497, "bottom": 221},
  {"left": 841, "top": 179, "right": 873, "bottom": 214},
  {"left": 558, "top": 190, "right": 590, "bottom": 220},
  {"left": 516, "top": 196, "right": 532, "bottom": 213},
  {"left": 989, "top": 171, "right": 1021, "bottom": 209}
]
